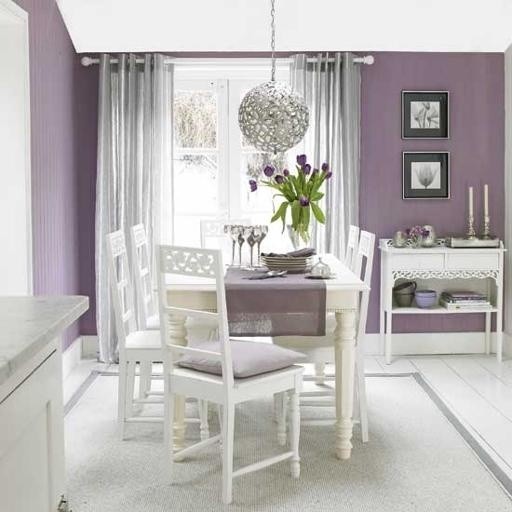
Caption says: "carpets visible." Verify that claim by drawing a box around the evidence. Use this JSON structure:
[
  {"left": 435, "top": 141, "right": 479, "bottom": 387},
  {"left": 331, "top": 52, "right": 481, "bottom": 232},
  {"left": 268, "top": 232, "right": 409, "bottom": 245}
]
[{"left": 62, "top": 371, "right": 512, "bottom": 512}]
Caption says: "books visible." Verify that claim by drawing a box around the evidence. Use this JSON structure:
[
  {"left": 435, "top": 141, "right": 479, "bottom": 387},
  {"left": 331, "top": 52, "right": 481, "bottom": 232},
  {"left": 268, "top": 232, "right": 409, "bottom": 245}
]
[{"left": 440, "top": 291, "right": 493, "bottom": 309}]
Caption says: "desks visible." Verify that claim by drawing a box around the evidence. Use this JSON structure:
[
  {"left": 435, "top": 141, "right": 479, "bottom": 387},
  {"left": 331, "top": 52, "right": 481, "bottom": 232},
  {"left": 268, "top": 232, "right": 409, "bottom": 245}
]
[{"left": 378, "top": 237, "right": 507, "bottom": 363}]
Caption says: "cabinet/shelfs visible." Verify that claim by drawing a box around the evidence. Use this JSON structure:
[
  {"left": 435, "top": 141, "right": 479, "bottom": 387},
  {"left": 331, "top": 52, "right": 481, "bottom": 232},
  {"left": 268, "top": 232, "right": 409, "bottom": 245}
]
[{"left": 0, "top": 294, "right": 90, "bottom": 512}]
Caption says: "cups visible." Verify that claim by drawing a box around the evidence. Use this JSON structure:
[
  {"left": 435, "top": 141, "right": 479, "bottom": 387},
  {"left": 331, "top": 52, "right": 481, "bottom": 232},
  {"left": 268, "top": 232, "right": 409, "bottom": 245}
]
[
  {"left": 393, "top": 281, "right": 417, "bottom": 294},
  {"left": 393, "top": 231, "right": 407, "bottom": 248},
  {"left": 393, "top": 294, "right": 414, "bottom": 307}
]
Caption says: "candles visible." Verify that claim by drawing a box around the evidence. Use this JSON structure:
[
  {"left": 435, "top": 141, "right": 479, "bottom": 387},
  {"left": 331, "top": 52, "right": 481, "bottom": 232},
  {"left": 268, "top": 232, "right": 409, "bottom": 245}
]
[
  {"left": 468, "top": 186, "right": 473, "bottom": 218},
  {"left": 483, "top": 184, "right": 488, "bottom": 218}
]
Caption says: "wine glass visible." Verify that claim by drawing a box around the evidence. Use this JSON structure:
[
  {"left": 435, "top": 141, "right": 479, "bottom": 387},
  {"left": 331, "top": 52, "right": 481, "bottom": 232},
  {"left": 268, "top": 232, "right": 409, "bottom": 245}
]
[{"left": 224, "top": 225, "right": 268, "bottom": 270}]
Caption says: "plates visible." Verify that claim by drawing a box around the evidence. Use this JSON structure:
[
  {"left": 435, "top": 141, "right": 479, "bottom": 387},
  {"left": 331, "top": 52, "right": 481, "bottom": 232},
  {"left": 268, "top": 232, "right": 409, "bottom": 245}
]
[
  {"left": 305, "top": 274, "right": 331, "bottom": 279},
  {"left": 262, "top": 256, "right": 308, "bottom": 273}
]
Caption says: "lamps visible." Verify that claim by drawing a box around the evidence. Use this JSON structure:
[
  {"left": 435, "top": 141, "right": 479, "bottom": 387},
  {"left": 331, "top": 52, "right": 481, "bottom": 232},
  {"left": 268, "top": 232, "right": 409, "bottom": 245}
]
[{"left": 238, "top": 0, "right": 309, "bottom": 154}]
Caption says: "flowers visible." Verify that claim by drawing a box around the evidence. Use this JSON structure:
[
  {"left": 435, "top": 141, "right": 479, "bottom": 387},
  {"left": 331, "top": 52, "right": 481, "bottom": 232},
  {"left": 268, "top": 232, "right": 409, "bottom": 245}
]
[{"left": 250, "top": 155, "right": 332, "bottom": 233}]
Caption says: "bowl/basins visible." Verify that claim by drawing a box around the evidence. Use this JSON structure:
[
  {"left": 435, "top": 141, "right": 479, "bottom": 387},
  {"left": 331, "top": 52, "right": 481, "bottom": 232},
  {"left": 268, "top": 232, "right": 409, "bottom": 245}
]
[
  {"left": 414, "top": 296, "right": 435, "bottom": 308},
  {"left": 415, "top": 290, "right": 436, "bottom": 297}
]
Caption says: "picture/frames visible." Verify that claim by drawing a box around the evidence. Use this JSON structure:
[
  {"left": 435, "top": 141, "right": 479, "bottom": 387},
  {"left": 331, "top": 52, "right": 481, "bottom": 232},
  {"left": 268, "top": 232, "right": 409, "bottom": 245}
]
[
  {"left": 402, "top": 151, "right": 450, "bottom": 202},
  {"left": 400, "top": 89, "right": 450, "bottom": 142}
]
